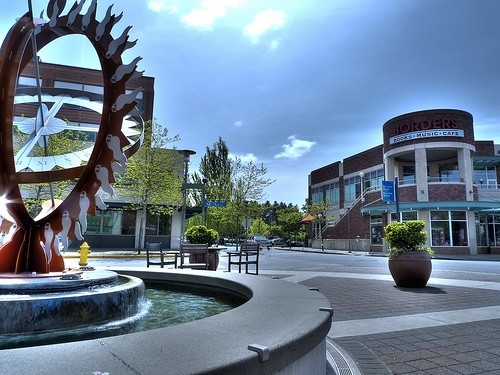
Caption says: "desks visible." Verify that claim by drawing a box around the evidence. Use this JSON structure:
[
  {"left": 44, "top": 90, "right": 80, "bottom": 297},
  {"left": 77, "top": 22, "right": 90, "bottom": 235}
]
[{"left": 206, "top": 247, "right": 226, "bottom": 270}]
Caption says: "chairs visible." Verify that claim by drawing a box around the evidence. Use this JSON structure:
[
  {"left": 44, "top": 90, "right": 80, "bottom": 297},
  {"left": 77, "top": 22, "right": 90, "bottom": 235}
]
[
  {"left": 180, "top": 243, "right": 209, "bottom": 270},
  {"left": 147, "top": 241, "right": 179, "bottom": 269},
  {"left": 227, "top": 242, "right": 260, "bottom": 275}
]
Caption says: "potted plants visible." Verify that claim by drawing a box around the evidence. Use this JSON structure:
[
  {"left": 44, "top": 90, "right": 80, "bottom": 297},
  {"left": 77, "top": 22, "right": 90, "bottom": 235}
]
[
  {"left": 185, "top": 225, "right": 219, "bottom": 271},
  {"left": 381, "top": 220, "right": 434, "bottom": 289}
]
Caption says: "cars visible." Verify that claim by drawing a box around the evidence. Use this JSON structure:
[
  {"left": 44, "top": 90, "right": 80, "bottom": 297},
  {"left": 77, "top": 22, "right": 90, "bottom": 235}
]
[{"left": 224, "top": 235, "right": 305, "bottom": 246}]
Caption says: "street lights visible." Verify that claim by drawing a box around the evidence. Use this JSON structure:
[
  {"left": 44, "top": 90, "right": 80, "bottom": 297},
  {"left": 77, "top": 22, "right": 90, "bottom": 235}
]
[
  {"left": 177, "top": 149, "right": 196, "bottom": 252},
  {"left": 318, "top": 213, "right": 324, "bottom": 250}
]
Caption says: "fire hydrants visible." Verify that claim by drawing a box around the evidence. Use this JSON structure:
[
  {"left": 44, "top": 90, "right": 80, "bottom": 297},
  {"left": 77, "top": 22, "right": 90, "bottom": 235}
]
[{"left": 77, "top": 242, "right": 91, "bottom": 266}]
